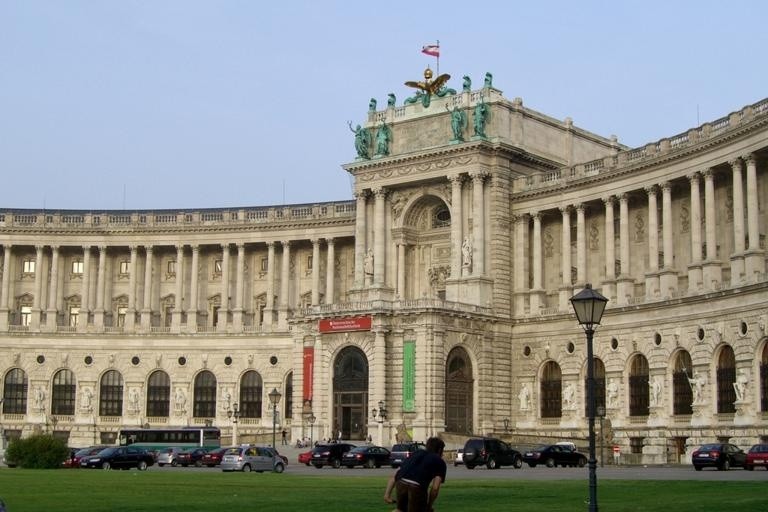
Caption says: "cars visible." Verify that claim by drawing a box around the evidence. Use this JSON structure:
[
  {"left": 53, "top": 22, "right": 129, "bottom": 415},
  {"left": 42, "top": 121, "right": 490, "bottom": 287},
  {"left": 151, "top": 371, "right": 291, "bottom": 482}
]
[
  {"left": 157, "top": 446, "right": 185, "bottom": 467},
  {"left": 453, "top": 447, "right": 465, "bottom": 467},
  {"left": 342, "top": 446, "right": 392, "bottom": 469},
  {"left": 176, "top": 448, "right": 209, "bottom": 467},
  {"left": 745, "top": 444, "right": 768, "bottom": 470},
  {"left": 79, "top": 447, "right": 156, "bottom": 471},
  {"left": 266, "top": 448, "right": 289, "bottom": 466},
  {"left": 298, "top": 445, "right": 328, "bottom": 466},
  {"left": 64, "top": 447, "right": 105, "bottom": 468},
  {"left": 201, "top": 447, "right": 229, "bottom": 468},
  {"left": 692, "top": 443, "right": 750, "bottom": 470},
  {"left": 523, "top": 445, "right": 587, "bottom": 468},
  {"left": 220, "top": 446, "right": 285, "bottom": 475},
  {"left": 554, "top": 442, "right": 576, "bottom": 455}
]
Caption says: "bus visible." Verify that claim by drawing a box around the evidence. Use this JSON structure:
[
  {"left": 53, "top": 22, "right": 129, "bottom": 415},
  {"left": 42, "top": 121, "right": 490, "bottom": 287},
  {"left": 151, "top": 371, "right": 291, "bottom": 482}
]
[{"left": 117, "top": 426, "right": 220, "bottom": 452}]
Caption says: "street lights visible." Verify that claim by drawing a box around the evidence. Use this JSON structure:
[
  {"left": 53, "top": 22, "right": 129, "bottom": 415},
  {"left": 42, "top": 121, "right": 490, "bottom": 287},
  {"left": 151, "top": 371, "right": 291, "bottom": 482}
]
[
  {"left": 504, "top": 416, "right": 509, "bottom": 435},
  {"left": 268, "top": 387, "right": 282, "bottom": 473},
  {"left": 308, "top": 412, "right": 316, "bottom": 451},
  {"left": 372, "top": 399, "right": 388, "bottom": 447},
  {"left": 227, "top": 401, "right": 243, "bottom": 447},
  {"left": 568, "top": 284, "right": 609, "bottom": 512}
]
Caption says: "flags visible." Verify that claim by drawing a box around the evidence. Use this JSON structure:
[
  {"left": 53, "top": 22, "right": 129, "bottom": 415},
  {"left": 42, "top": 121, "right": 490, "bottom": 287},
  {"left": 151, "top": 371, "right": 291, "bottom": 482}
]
[{"left": 421, "top": 44, "right": 439, "bottom": 57}]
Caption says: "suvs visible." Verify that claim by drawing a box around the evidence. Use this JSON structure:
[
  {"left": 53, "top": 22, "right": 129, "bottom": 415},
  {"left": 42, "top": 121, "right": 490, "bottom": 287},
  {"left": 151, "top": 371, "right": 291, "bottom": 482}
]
[
  {"left": 310, "top": 444, "right": 357, "bottom": 469},
  {"left": 462, "top": 438, "right": 522, "bottom": 469},
  {"left": 389, "top": 442, "right": 426, "bottom": 468}
]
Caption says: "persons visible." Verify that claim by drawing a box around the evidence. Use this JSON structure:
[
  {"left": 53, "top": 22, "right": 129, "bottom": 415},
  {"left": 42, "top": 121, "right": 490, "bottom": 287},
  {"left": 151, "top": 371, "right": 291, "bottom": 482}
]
[
  {"left": 295, "top": 428, "right": 372, "bottom": 448},
  {"left": 70, "top": 447, "right": 81, "bottom": 470},
  {"left": 347, "top": 119, "right": 370, "bottom": 159},
  {"left": 364, "top": 248, "right": 375, "bottom": 275},
  {"left": 376, "top": 123, "right": 390, "bottom": 155},
  {"left": 33, "top": 385, "right": 232, "bottom": 413},
  {"left": 470, "top": 102, "right": 487, "bottom": 138},
  {"left": 383, "top": 436, "right": 446, "bottom": 511},
  {"left": 282, "top": 427, "right": 289, "bottom": 445},
  {"left": 445, "top": 105, "right": 465, "bottom": 140},
  {"left": 461, "top": 236, "right": 473, "bottom": 265},
  {"left": 517, "top": 368, "right": 749, "bottom": 411}
]
